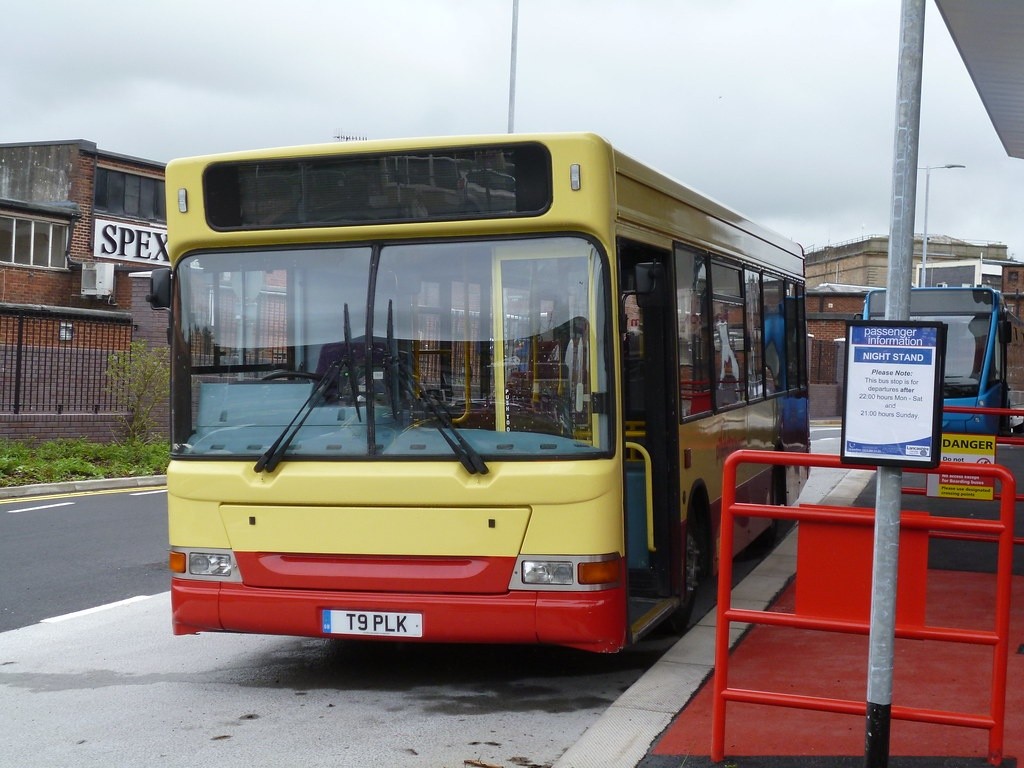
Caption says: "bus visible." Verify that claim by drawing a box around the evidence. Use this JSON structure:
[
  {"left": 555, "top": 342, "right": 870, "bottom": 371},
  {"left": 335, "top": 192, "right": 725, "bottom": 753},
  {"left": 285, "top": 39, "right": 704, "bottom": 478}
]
[
  {"left": 853, "top": 283, "right": 1024, "bottom": 437},
  {"left": 143, "top": 133, "right": 813, "bottom": 647}
]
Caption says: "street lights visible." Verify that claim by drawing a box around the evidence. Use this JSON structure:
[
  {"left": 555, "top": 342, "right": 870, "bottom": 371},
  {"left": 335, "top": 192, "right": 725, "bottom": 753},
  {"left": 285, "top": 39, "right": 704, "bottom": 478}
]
[{"left": 913, "top": 164, "right": 966, "bottom": 288}]
